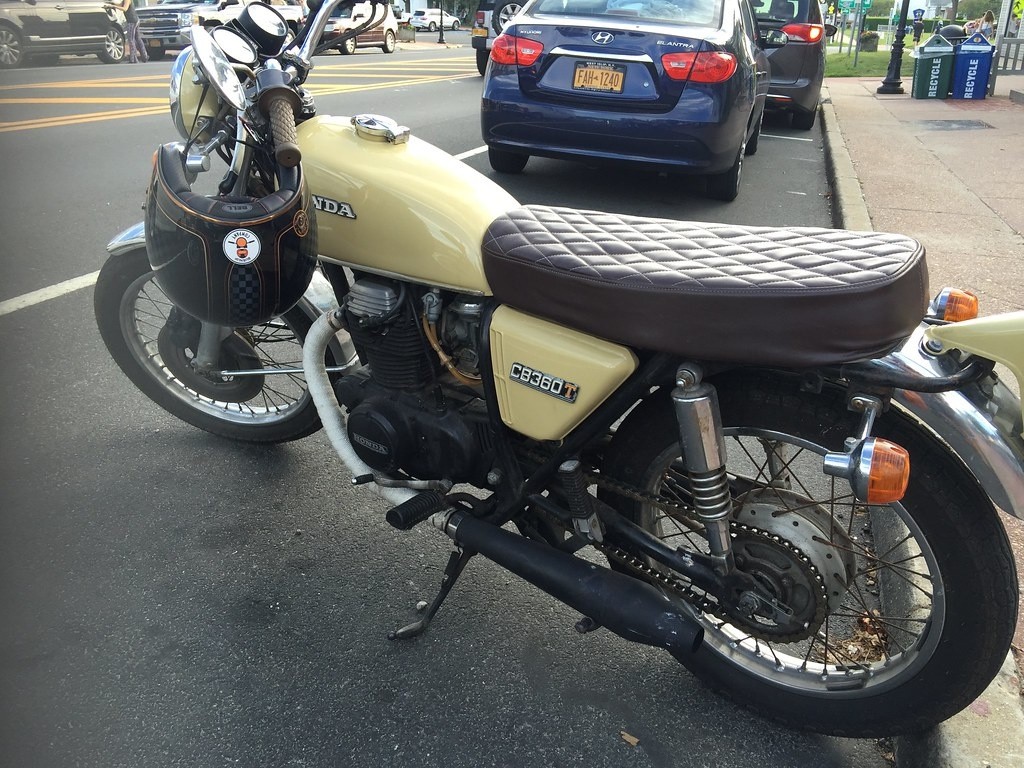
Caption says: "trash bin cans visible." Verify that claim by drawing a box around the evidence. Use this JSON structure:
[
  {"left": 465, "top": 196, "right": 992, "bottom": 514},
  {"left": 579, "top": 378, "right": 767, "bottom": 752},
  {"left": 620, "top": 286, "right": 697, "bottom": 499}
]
[
  {"left": 910, "top": 34, "right": 953, "bottom": 99},
  {"left": 952, "top": 32, "right": 996, "bottom": 100}
]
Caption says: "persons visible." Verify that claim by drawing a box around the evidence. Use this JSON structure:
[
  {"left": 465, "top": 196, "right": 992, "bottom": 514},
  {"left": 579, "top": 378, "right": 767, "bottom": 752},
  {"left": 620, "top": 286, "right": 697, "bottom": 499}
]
[
  {"left": 103, "top": 0, "right": 150, "bottom": 65},
  {"left": 913, "top": 10, "right": 1020, "bottom": 51}
]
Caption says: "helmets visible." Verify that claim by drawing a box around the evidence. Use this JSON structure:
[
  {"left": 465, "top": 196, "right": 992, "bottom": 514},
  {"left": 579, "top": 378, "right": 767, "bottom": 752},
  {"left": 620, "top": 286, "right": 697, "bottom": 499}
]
[{"left": 143, "top": 145, "right": 318, "bottom": 328}]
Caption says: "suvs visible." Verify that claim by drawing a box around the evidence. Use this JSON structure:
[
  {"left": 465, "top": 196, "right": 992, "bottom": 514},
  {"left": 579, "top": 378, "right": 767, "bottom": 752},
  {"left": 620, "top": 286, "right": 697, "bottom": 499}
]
[
  {"left": 136, "top": 0, "right": 304, "bottom": 61},
  {"left": 471, "top": 0, "right": 528, "bottom": 78}
]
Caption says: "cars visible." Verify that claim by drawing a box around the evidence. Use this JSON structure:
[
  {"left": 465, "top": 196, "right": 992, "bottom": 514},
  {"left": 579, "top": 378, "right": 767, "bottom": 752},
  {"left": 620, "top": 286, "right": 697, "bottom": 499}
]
[
  {"left": 0, "top": 0, "right": 128, "bottom": 70},
  {"left": 299, "top": 1, "right": 398, "bottom": 55},
  {"left": 750, "top": 0, "right": 839, "bottom": 131},
  {"left": 409, "top": 9, "right": 461, "bottom": 33},
  {"left": 481, "top": 1, "right": 791, "bottom": 202}
]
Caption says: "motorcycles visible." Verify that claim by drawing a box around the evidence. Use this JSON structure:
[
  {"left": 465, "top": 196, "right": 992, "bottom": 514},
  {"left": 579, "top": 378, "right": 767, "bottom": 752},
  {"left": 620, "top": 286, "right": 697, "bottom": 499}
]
[{"left": 94, "top": 0, "right": 1024, "bottom": 738}]
